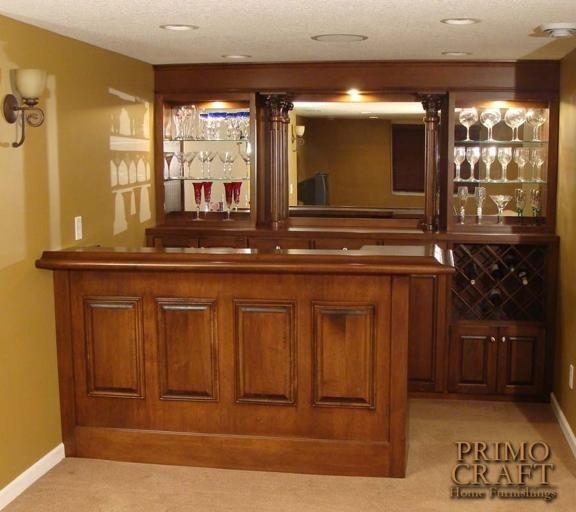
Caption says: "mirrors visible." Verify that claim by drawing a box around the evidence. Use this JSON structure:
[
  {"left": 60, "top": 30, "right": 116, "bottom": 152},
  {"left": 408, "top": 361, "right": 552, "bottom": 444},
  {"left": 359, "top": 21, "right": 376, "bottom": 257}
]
[{"left": 268, "top": 88, "right": 439, "bottom": 230}]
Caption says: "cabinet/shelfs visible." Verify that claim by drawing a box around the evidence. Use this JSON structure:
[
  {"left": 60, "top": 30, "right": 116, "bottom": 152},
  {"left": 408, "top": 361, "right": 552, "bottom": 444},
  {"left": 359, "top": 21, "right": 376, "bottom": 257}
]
[
  {"left": 454, "top": 140, "right": 548, "bottom": 185},
  {"left": 162, "top": 137, "right": 250, "bottom": 182},
  {"left": 449, "top": 321, "right": 553, "bottom": 402},
  {"left": 449, "top": 242, "right": 546, "bottom": 322}
]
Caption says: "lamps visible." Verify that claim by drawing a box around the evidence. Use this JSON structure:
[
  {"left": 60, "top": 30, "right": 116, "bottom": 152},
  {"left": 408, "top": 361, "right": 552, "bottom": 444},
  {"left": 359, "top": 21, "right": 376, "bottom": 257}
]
[
  {"left": 288, "top": 123, "right": 305, "bottom": 153},
  {"left": 3, "top": 69, "right": 46, "bottom": 146}
]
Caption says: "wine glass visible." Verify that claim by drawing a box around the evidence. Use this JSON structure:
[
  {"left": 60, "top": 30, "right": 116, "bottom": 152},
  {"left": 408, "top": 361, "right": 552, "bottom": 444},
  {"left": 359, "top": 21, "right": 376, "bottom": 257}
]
[
  {"left": 239, "top": 142, "right": 253, "bottom": 180},
  {"left": 504, "top": 110, "right": 515, "bottom": 143},
  {"left": 183, "top": 151, "right": 198, "bottom": 179},
  {"left": 111, "top": 187, "right": 152, "bottom": 235},
  {"left": 172, "top": 104, "right": 249, "bottom": 141},
  {"left": 110, "top": 149, "right": 152, "bottom": 186},
  {"left": 196, "top": 151, "right": 213, "bottom": 180},
  {"left": 479, "top": 113, "right": 490, "bottom": 142},
  {"left": 204, "top": 152, "right": 218, "bottom": 180},
  {"left": 453, "top": 147, "right": 545, "bottom": 183},
  {"left": 458, "top": 108, "right": 478, "bottom": 141},
  {"left": 218, "top": 152, "right": 235, "bottom": 179},
  {"left": 109, "top": 93, "right": 147, "bottom": 139},
  {"left": 526, "top": 109, "right": 548, "bottom": 141},
  {"left": 508, "top": 108, "right": 526, "bottom": 142},
  {"left": 222, "top": 183, "right": 236, "bottom": 223},
  {"left": 457, "top": 187, "right": 546, "bottom": 227},
  {"left": 203, "top": 182, "right": 214, "bottom": 214},
  {"left": 226, "top": 154, "right": 239, "bottom": 178},
  {"left": 191, "top": 183, "right": 204, "bottom": 223},
  {"left": 174, "top": 152, "right": 190, "bottom": 179},
  {"left": 481, "top": 109, "right": 501, "bottom": 142},
  {"left": 234, "top": 181, "right": 242, "bottom": 213}
]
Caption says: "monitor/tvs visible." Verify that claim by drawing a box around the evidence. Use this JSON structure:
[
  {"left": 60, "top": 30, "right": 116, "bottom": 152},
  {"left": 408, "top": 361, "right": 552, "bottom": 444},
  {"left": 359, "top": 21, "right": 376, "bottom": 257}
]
[{"left": 297, "top": 173, "right": 328, "bottom": 205}]
